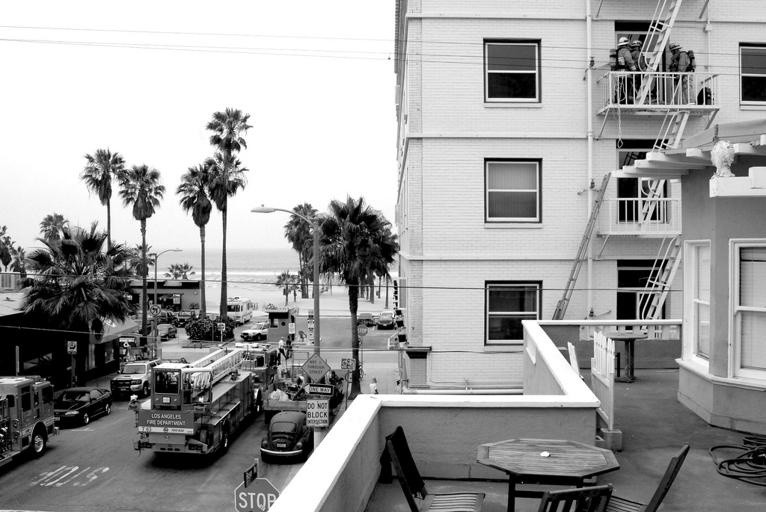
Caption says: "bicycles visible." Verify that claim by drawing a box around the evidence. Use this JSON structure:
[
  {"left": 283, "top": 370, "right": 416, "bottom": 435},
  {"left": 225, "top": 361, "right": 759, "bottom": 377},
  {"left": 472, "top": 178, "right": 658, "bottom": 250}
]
[{"left": 345, "top": 364, "right": 364, "bottom": 383}]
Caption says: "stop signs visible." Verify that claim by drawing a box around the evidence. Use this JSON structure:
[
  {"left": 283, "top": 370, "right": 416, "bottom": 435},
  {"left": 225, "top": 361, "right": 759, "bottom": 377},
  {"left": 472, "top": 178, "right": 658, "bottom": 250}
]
[{"left": 234, "top": 477, "right": 281, "bottom": 512}]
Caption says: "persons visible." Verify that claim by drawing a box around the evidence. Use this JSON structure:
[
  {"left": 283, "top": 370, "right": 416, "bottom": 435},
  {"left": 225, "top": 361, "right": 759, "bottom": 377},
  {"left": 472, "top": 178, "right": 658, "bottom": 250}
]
[
  {"left": 627, "top": 39, "right": 650, "bottom": 105},
  {"left": 370, "top": 378, "right": 379, "bottom": 395},
  {"left": 613, "top": 36, "right": 637, "bottom": 104},
  {"left": 285, "top": 336, "right": 293, "bottom": 359},
  {"left": 278, "top": 336, "right": 289, "bottom": 361},
  {"left": 667, "top": 42, "right": 694, "bottom": 104}
]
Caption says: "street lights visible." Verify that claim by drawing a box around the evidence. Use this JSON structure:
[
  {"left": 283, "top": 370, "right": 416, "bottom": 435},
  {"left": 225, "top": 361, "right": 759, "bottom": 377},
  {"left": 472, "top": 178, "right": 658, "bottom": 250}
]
[
  {"left": 154, "top": 249, "right": 183, "bottom": 359},
  {"left": 252, "top": 206, "right": 320, "bottom": 357}
]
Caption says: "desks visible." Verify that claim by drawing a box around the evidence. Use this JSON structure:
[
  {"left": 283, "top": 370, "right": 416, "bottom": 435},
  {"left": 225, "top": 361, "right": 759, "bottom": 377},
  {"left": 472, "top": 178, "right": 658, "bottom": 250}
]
[
  {"left": 589, "top": 330, "right": 649, "bottom": 383},
  {"left": 474, "top": 436, "right": 621, "bottom": 512}
]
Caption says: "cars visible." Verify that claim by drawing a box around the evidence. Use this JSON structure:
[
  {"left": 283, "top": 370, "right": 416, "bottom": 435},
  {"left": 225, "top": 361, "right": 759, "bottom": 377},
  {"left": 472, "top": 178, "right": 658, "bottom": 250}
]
[
  {"left": 260, "top": 411, "right": 314, "bottom": 462},
  {"left": 54, "top": 386, "right": 112, "bottom": 425},
  {"left": 157, "top": 310, "right": 196, "bottom": 340},
  {"left": 357, "top": 313, "right": 394, "bottom": 328},
  {"left": 240, "top": 322, "right": 269, "bottom": 341}
]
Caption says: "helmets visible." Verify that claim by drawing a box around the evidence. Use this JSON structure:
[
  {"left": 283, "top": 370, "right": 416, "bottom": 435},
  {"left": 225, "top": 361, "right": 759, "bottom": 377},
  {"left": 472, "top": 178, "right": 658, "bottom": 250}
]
[
  {"left": 668, "top": 41, "right": 683, "bottom": 52},
  {"left": 616, "top": 36, "right": 629, "bottom": 47},
  {"left": 630, "top": 40, "right": 644, "bottom": 48}
]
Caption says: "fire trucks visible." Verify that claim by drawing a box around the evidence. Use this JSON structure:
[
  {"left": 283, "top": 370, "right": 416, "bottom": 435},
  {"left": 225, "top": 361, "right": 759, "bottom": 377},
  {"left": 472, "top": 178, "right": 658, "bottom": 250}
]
[
  {"left": 0, "top": 375, "right": 61, "bottom": 464},
  {"left": 128, "top": 344, "right": 281, "bottom": 456}
]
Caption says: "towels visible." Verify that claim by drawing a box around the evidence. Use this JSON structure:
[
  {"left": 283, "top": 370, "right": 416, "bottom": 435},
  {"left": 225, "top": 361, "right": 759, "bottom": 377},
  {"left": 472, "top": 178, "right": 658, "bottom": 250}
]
[{"left": 379, "top": 427, "right": 425, "bottom": 494}]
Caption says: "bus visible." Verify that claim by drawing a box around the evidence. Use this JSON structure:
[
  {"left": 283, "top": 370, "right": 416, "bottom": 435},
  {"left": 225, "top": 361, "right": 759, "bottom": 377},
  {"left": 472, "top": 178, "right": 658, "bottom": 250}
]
[{"left": 227, "top": 297, "right": 253, "bottom": 324}]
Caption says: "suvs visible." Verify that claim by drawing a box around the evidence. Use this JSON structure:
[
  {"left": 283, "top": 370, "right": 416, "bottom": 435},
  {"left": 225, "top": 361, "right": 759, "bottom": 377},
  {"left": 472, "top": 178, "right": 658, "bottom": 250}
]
[{"left": 110, "top": 358, "right": 162, "bottom": 396}]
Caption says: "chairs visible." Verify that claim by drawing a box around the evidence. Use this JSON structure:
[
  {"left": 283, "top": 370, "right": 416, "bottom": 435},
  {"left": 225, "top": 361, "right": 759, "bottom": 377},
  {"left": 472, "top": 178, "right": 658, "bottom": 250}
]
[
  {"left": 386, "top": 427, "right": 486, "bottom": 512},
  {"left": 537, "top": 444, "right": 691, "bottom": 512}
]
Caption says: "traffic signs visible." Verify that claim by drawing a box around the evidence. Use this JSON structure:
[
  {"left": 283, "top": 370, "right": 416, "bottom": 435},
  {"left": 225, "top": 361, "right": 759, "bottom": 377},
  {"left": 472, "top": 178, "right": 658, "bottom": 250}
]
[
  {"left": 243, "top": 463, "right": 257, "bottom": 487},
  {"left": 303, "top": 385, "right": 334, "bottom": 397}
]
